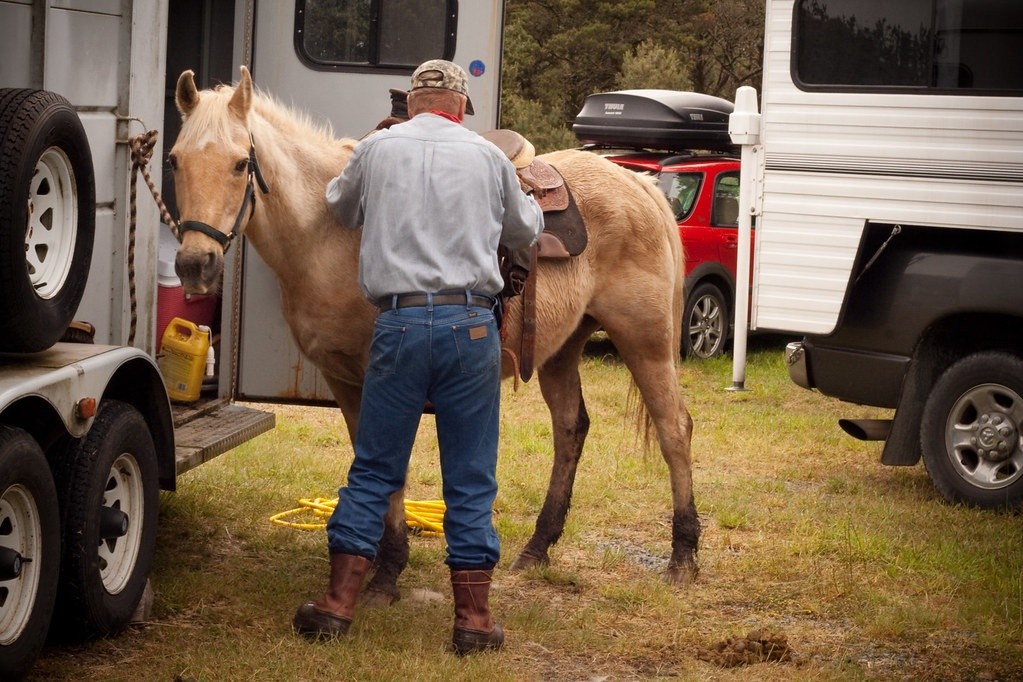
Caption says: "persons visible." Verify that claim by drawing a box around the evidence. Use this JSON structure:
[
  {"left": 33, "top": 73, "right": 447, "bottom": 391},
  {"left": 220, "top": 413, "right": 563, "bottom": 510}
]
[{"left": 291, "top": 57, "right": 546, "bottom": 658}]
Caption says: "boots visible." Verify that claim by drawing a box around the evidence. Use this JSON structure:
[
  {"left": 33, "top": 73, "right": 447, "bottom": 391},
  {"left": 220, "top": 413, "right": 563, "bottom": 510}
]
[
  {"left": 449, "top": 566, "right": 505, "bottom": 655},
  {"left": 294, "top": 551, "right": 375, "bottom": 639}
]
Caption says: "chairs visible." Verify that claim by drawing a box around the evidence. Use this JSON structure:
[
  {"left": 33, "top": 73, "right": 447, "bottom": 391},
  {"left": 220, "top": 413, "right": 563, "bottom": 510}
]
[{"left": 715, "top": 190, "right": 740, "bottom": 225}]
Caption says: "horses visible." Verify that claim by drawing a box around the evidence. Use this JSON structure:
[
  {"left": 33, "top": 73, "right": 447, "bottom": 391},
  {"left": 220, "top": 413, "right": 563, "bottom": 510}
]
[{"left": 166, "top": 65, "right": 702, "bottom": 590}]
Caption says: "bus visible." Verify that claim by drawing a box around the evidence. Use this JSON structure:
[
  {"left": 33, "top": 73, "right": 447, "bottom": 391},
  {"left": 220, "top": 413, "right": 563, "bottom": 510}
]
[
  {"left": 718, "top": 1, "right": 1023, "bottom": 520},
  {"left": 0, "top": 0, "right": 509, "bottom": 679}
]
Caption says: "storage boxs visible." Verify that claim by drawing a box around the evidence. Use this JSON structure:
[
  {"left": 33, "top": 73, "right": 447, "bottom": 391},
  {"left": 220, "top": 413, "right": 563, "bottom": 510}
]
[{"left": 154, "top": 220, "right": 216, "bottom": 355}]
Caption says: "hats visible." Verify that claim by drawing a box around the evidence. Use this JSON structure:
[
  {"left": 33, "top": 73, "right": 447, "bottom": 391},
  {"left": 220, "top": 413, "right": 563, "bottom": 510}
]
[{"left": 408, "top": 59, "right": 475, "bottom": 116}]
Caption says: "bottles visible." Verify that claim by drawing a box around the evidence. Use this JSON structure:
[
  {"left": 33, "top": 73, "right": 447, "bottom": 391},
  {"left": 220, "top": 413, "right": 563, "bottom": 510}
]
[{"left": 157, "top": 319, "right": 211, "bottom": 405}]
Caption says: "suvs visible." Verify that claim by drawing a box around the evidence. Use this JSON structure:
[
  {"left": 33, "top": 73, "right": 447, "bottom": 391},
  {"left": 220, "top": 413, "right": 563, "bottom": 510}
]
[{"left": 571, "top": 89, "right": 754, "bottom": 365}]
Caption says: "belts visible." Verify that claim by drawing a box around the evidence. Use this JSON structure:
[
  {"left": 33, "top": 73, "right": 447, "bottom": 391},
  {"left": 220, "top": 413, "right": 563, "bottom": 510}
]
[{"left": 378, "top": 292, "right": 496, "bottom": 314}]
